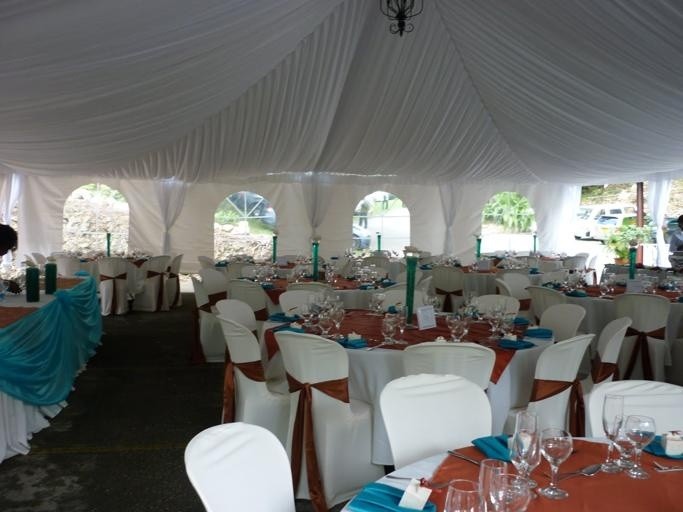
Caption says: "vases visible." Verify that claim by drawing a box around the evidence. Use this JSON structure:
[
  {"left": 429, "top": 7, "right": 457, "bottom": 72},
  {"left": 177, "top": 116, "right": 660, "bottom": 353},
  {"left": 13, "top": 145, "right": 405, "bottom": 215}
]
[{"left": 628, "top": 247, "right": 636, "bottom": 278}]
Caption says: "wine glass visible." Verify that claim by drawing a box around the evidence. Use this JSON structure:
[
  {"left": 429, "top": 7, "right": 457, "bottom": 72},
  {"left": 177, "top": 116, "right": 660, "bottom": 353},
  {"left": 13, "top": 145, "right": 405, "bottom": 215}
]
[
  {"left": 299, "top": 291, "right": 516, "bottom": 353},
  {"left": 252, "top": 263, "right": 385, "bottom": 288},
  {"left": 443, "top": 394, "right": 656, "bottom": 512}
]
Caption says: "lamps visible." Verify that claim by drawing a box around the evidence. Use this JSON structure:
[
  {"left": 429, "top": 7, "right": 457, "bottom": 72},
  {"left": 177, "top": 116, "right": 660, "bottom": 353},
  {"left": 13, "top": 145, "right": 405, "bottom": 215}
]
[{"left": 380, "top": 0, "right": 423, "bottom": 38}]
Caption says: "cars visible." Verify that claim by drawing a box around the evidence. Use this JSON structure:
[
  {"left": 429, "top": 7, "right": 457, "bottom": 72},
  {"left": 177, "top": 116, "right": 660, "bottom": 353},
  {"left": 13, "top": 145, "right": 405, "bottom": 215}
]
[{"left": 233, "top": 191, "right": 372, "bottom": 251}]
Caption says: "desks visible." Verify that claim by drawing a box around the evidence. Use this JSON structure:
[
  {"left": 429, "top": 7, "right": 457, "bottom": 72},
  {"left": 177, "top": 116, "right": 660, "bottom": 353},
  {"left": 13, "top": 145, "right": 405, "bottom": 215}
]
[
  {"left": 1, "top": 253, "right": 104, "bottom": 463},
  {"left": 338, "top": 435, "right": 683, "bottom": 512}
]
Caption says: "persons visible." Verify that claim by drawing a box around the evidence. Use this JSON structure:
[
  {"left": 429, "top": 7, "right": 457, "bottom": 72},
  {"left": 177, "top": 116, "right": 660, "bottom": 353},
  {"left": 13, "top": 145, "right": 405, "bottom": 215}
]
[
  {"left": 0, "top": 223, "right": 22, "bottom": 296},
  {"left": 360, "top": 200, "right": 370, "bottom": 228},
  {"left": 668, "top": 214, "right": 683, "bottom": 252}
]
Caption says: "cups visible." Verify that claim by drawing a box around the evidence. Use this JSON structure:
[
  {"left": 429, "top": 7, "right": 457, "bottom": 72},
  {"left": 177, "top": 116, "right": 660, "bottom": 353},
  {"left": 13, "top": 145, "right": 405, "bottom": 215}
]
[{"left": 598, "top": 272, "right": 616, "bottom": 298}]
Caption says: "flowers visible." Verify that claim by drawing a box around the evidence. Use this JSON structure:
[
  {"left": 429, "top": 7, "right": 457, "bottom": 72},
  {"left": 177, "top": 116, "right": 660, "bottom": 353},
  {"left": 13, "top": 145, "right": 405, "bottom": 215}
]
[{"left": 611, "top": 224, "right": 655, "bottom": 249}]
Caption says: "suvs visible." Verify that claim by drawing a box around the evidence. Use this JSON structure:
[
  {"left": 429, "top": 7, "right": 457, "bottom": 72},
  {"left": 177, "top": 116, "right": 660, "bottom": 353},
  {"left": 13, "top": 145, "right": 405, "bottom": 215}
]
[{"left": 575, "top": 201, "right": 639, "bottom": 244}]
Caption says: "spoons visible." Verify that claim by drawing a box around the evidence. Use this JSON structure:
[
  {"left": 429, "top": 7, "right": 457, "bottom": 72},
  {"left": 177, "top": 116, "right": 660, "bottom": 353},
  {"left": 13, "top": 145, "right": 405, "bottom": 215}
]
[{"left": 386, "top": 474, "right": 450, "bottom": 489}]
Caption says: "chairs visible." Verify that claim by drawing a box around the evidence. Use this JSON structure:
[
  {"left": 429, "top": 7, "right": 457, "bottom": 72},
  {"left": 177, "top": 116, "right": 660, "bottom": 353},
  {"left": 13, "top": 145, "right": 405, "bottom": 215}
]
[
  {"left": 188, "top": 254, "right": 682, "bottom": 423},
  {"left": 215, "top": 314, "right": 291, "bottom": 449},
  {"left": 183, "top": 421, "right": 296, "bottom": 512},
  {"left": 96, "top": 253, "right": 185, "bottom": 315},
  {"left": 379, "top": 373, "right": 492, "bottom": 470},
  {"left": 589, "top": 379, "right": 683, "bottom": 438},
  {"left": 569, "top": 316, "right": 633, "bottom": 437},
  {"left": 505, "top": 334, "right": 595, "bottom": 439},
  {"left": 273, "top": 331, "right": 387, "bottom": 512}
]
